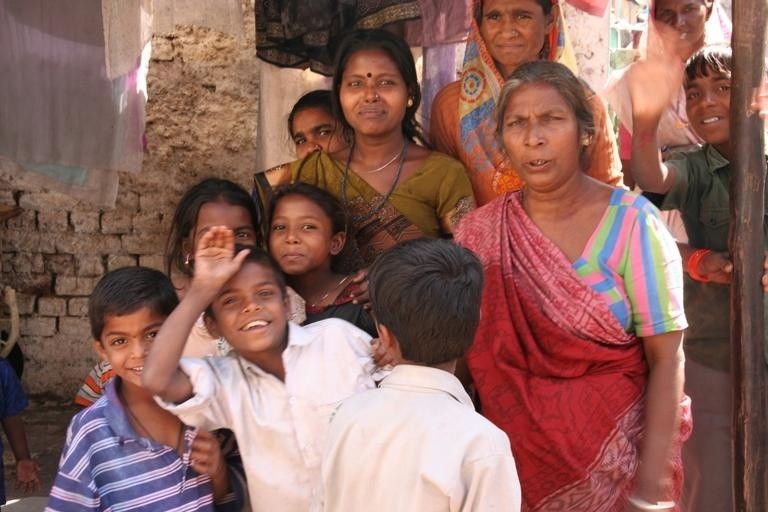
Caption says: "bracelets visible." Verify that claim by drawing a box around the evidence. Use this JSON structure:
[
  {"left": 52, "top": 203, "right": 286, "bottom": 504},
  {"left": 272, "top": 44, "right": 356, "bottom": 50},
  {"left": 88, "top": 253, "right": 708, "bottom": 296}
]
[
  {"left": 681, "top": 248, "right": 713, "bottom": 284},
  {"left": 621, "top": 492, "right": 678, "bottom": 511}
]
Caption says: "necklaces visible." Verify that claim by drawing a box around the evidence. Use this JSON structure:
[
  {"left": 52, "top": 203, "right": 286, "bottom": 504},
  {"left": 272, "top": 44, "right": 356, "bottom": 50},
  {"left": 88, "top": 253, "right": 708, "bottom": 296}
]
[
  {"left": 304, "top": 275, "right": 358, "bottom": 312},
  {"left": 349, "top": 142, "right": 407, "bottom": 177},
  {"left": 342, "top": 137, "right": 409, "bottom": 228},
  {"left": 113, "top": 382, "right": 193, "bottom": 459}
]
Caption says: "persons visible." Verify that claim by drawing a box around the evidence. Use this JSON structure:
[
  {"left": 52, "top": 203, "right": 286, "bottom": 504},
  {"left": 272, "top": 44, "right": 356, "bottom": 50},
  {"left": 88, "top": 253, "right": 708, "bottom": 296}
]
[
  {"left": 42, "top": 266, "right": 247, "bottom": 512},
  {"left": 287, "top": 89, "right": 356, "bottom": 160},
  {"left": 429, "top": 0, "right": 768, "bottom": 297},
  {"left": 608, "top": 2, "right": 717, "bottom": 244},
  {"left": 0, "top": 360, "right": 45, "bottom": 512},
  {"left": 451, "top": 59, "right": 692, "bottom": 512},
  {"left": 73, "top": 361, "right": 117, "bottom": 408},
  {"left": 264, "top": 183, "right": 397, "bottom": 366},
  {"left": 252, "top": 28, "right": 476, "bottom": 312},
  {"left": 312, "top": 237, "right": 522, "bottom": 512},
  {"left": 626, "top": 36, "right": 767, "bottom": 511},
  {"left": 141, "top": 224, "right": 397, "bottom": 512},
  {"left": 164, "top": 177, "right": 308, "bottom": 358}
]
